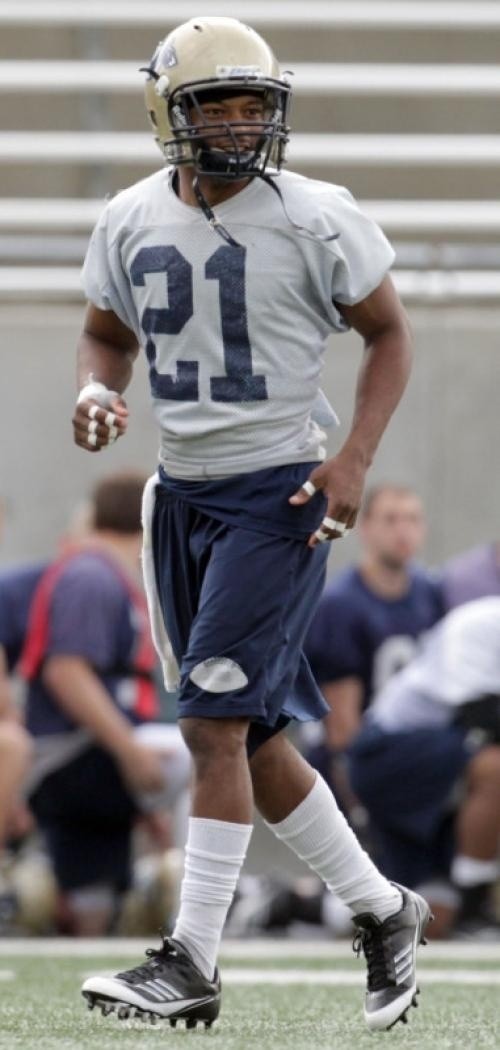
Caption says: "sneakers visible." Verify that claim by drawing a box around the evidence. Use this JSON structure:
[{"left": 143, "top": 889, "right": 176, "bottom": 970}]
[
  {"left": 346, "top": 876, "right": 434, "bottom": 1037},
  {"left": 80, "top": 935, "right": 224, "bottom": 1031}
]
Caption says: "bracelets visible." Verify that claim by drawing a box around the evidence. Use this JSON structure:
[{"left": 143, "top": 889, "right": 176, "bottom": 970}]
[{"left": 77, "top": 381, "right": 109, "bottom": 402}]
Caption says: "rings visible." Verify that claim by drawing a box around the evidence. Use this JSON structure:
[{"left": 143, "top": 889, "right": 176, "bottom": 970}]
[
  {"left": 86, "top": 434, "right": 98, "bottom": 448},
  {"left": 108, "top": 439, "right": 116, "bottom": 446},
  {"left": 108, "top": 427, "right": 118, "bottom": 437},
  {"left": 86, "top": 420, "right": 98, "bottom": 432},
  {"left": 104, "top": 411, "right": 115, "bottom": 426},
  {"left": 89, "top": 405, "right": 100, "bottom": 420}
]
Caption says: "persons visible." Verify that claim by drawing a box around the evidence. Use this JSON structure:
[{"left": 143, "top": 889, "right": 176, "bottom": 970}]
[
  {"left": 2, "top": 474, "right": 500, "bottom": 940},
  {"left": 68, "top": 14, "right": 431, "bottom": 1032}
]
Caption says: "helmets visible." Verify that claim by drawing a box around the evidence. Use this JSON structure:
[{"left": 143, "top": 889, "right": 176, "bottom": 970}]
[{"left": 141, "top": 14, "right": 293, "bottom": 165}]
